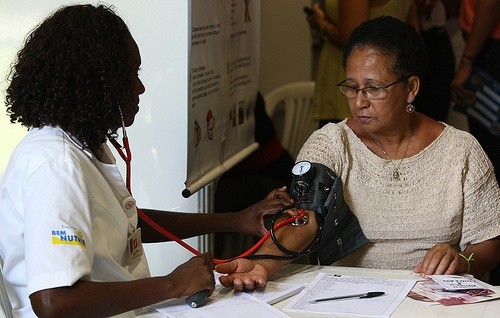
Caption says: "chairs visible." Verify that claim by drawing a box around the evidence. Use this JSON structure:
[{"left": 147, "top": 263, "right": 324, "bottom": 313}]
[{"left": 262, "top": 80, "right": 317, "bottom": 160}]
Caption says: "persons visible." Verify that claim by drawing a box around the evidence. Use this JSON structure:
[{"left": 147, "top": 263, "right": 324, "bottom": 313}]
[
  {"left": 215, "top": 16, "right": 500, "bottom": 291},
  {"left": 0, "top": 3, "right": 302, "bottom": 318},
  {"left": 304, "top": 0, "right": 500, "bottom": 185}
]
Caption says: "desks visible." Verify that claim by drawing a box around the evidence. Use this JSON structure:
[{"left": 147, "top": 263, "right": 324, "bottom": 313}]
[{"left": 111, "top": 262, "right": 500, "bottom": 318}]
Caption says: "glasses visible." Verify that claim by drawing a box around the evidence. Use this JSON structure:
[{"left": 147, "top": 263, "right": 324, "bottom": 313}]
[{"left": 337, "top": 77, "right": 406, "bottom": 100}]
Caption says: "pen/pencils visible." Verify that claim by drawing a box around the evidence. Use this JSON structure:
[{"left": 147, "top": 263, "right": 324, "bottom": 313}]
[{"left": 308, "top": 291, "right": 385, "bottom": 304}]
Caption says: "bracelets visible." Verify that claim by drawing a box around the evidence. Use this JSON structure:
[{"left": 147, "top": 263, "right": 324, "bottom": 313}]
[{"left": 459, "top": 252, "right": 475, "bottom": 274}]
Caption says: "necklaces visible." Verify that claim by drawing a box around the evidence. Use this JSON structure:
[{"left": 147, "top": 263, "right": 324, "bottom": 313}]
[{"left": 371, "top": 126, "right": 413, "bottom": 178}]
